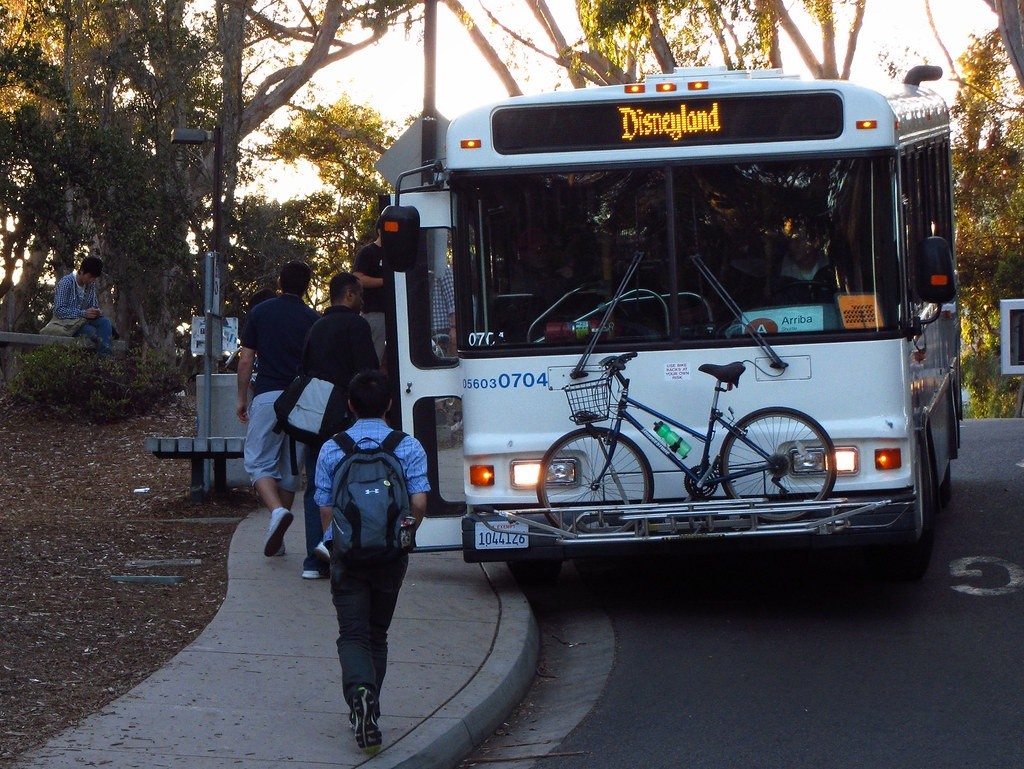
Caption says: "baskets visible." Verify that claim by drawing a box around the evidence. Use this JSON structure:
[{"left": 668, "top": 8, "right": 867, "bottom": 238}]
[{"left": 563, "top": 378, "right": 613, "bottom": 425}]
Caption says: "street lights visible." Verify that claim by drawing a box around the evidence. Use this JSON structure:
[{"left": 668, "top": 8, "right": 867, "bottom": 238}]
[{"left": 169, "top": 126, "right": 229, "bottom": 495}]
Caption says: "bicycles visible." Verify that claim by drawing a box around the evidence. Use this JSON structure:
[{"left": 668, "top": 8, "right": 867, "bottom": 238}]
[{"left": 537, "top": 351, "right": 837, "bottom": 535}]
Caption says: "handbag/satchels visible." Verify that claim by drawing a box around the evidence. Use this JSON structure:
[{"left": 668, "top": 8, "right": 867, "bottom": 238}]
[
  {"left": 39, "top": 314, "right": 87, "bottom": 337},
  {"left": 273, "top": 374, "right": 355, "bottom": 447}
]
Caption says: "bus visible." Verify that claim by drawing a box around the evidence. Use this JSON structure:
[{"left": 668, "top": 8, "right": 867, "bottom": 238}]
[{"left": 379, "top": 64, "right": 963, "bottom": 581}]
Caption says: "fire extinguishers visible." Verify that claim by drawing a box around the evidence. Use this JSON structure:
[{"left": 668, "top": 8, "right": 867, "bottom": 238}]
[{"left": 545, "top": 321, "right": 614, "bottom": 346}]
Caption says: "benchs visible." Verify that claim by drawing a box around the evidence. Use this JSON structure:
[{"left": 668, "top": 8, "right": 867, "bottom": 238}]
[
  {"left": 146, "top": 436, "right": 248, "bottom": 507},
  {"left": 0, "top": 330, "right": 125, "bottom": 376}
]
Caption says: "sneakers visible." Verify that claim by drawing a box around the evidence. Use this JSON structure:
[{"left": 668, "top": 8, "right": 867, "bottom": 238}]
[
  {"left": 350, "top": 686, "right": 381, "bottom": 755},
  {"left": 303, "top": 557, "right": 329, "bottom": 578},
  {"left": 264, "top": 508, "right": 293, "bottom": 556},
  {"left": 314, "top": 542, "right": 332, "bottom": 559}
]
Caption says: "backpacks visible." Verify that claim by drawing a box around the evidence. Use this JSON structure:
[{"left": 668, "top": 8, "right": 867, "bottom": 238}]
[{"left": 331, "top": 429, "right": 416, "bottom": 567}]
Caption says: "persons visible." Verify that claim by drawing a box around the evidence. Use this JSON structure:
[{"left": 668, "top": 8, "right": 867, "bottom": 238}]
[
  {"left": 302, "top": 272, "right": 381, "bottom": 579},
  {"left": 53, "top": 256, "right": 113, "bottom": 359},
  {"left": 236, "top": 263, "right": 319, "bottom": 557},
  {"left": 786, "top": 234, "right": 823, "bottom": 304},
  {"left": 429, "top": 266, "right": 458, "bottom": 413},
  {"left": 314, "top": 370, "right": 431, "bottom": 754},
  {"left": 511, "top": 228, "right": 544, "bottom": 322},
  {"left": 352, "top": 217, "right": 385, "bottom": 360}
]
